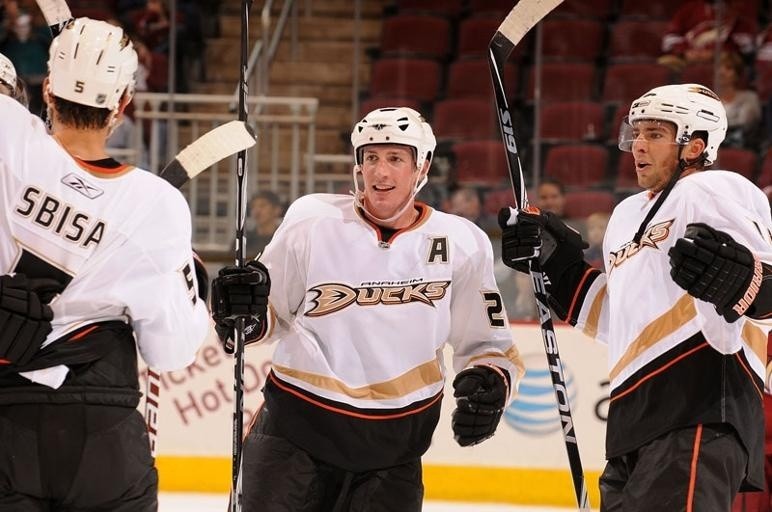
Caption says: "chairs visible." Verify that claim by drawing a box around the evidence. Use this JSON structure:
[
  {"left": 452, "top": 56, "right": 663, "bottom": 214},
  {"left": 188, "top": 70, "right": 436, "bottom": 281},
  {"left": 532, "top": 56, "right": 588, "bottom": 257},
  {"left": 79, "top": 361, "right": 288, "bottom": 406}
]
[
  {"left": 339, "top": 0, "right": 772, "bottom": 219},
  {"left": 24, "top": 3, "right": 223, "bottom": 158}
]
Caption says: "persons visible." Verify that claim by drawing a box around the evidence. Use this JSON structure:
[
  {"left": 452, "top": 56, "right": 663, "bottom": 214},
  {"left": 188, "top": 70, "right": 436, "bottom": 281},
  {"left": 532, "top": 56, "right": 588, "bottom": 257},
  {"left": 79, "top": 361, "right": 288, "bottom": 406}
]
[
  {"left": 0, "top": 15, "right": 210, "bottom": 511},
  {"left": 211, "top": 105, "right": 526, "bottom": 511},
  {"left": 495, "top": 81, "right": 770, "bottom": 512}
]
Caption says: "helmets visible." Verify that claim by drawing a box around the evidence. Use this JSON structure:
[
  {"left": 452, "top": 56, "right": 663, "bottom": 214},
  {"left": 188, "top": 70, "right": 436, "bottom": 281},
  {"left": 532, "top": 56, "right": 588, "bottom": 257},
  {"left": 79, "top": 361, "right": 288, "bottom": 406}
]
[
  {"left": 348, "top": 107, "right": 437, "bottom": 222},
  {"left": 0, "top": 52, "right": 18, "bottom": 92},
  {"left": 47, "top": 17, "right": 139, "bottom": 110},
  {"left": 617, "top": 83, "right": 729, "bottom": 169}
]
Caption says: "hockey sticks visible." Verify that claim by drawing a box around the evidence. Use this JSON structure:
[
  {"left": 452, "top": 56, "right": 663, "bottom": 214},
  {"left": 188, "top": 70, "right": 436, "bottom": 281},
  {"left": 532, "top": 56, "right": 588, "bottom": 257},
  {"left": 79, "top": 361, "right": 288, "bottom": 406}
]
[
  {"left": 488, "top": 1, "right": 591, "bottom": 511},
  {"left": 159, "top": 119, "right": 256, "bottom": 189}
]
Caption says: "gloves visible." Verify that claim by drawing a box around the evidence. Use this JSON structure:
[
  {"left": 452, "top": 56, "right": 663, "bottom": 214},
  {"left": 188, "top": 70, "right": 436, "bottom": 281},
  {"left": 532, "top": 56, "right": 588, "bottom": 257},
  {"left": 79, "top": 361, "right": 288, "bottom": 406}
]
[
  {"left": 450, "top": 362, "right": 511, "bottom": 448},
  {"left": 2, "top": 273, "right": 61, "bottom": 364},
  {"left": 668, "top": 223, "right": 772, "bottom": 323},
  {"left": 210, "top": 260, "right": 272, "bottom": 330},
  {"left": 498, "top": 205, "right": 591, "bottom": 321}
]
